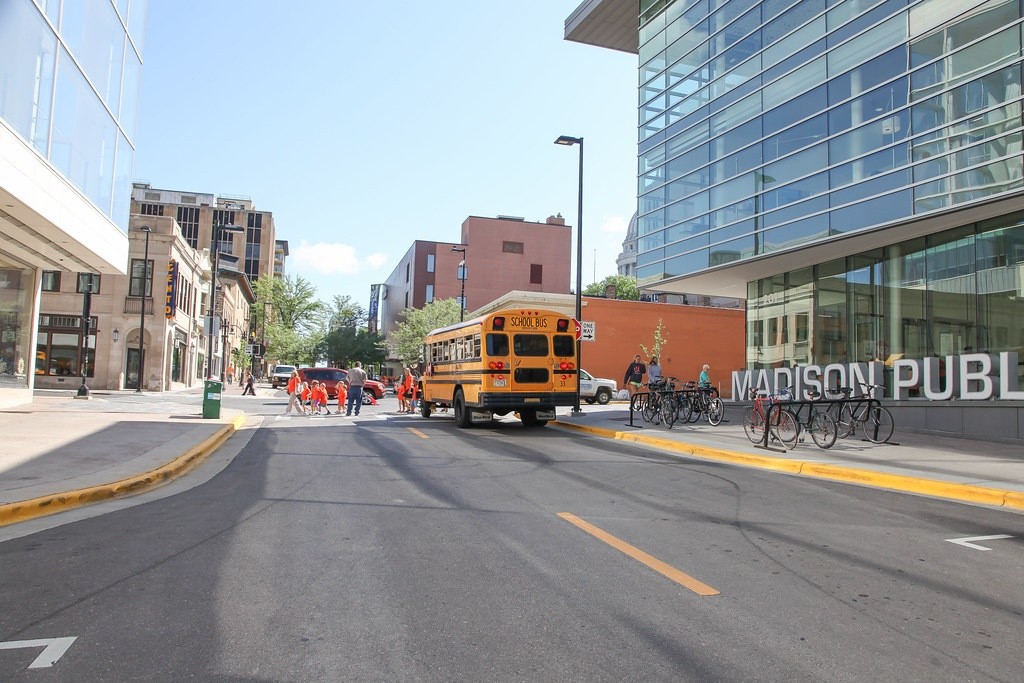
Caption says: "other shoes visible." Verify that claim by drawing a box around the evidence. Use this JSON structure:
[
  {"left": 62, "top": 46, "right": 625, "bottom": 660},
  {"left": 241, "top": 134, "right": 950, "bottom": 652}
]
[
  {"left": 397, "top": 409, "right": 449, "bottom": 415},
  {"left": 334, "top": 411, "right": 341, "bottom": 414},
  {"left": 283, "top": 407, "right": 332, "bottom": 416}
]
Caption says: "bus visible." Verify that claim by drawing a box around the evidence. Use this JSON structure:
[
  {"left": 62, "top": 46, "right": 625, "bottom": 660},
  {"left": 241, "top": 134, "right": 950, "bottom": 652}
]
[{"left": 410, "top": 309, "right": 578, "bottom": 427}]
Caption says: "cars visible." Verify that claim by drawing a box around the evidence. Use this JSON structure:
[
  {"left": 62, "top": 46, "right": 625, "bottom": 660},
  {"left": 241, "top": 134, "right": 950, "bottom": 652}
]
[{"left": 393, "top": 375, "right": 419, "bottom": 395}]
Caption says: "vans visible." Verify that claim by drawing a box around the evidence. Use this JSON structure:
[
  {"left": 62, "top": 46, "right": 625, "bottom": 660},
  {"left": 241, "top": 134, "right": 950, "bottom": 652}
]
[{"left": 272, "top": 364, "right": 296, "bottom": 389}]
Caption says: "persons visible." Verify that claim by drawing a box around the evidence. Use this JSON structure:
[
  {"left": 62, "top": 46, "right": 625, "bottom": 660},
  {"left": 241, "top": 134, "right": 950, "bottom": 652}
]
[
  {"left": 622, "top": 355, "right": 646, "bottom": 410},
  {"left": 298, "top": 382, "right": 312, "bottom": 414},
  {"left": 62, "top": 362, "right": 75, "bottom": 376},
  {"left": 309, "top": 380, "right": 331, "bottom": 416},
  {"left": 284, "top": 370, "right": 304, "bottom": 415},
  {"left": 393, "top": 367, "right": 419, "bottom": 414},
  {"left": 226, "top": 363, "right": 234, "bottom": 386},
  {"left": 334, "top": 381, "right": 347, "bottom": 415},
  {"left": 380, "top": 375, "right": 396, "bottom": 386},
  {"left": 241, "top": 370, "right": 257, "bottom": 396},
  {"left": 698, "top": 365, "right": 712, "bottom": 405},
  {"left": 648, "top": 357, "right": 662, "bottom": 411},
  {"left": 345, "top": 361, "right": 367, "bottom": 417}
]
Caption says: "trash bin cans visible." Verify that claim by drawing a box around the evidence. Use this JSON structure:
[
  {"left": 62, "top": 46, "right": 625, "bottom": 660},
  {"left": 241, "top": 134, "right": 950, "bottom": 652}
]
[{"left": 203, "top": 380, "right": 223, "bottom": 419}]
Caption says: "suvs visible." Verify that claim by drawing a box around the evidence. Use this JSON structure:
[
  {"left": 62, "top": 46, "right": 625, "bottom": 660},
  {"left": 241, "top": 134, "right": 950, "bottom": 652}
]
[
  {"left": 579, "top": 369, "right": 618, "bottom": 404},
  {"left": 287, "top": 367, "right": 386, "bottom": 405}
]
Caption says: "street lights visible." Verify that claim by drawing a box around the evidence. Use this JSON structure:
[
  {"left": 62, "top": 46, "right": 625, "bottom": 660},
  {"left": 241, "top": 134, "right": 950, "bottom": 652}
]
[
  {"left": 554, "top": 136, "right": 584, "bottom": 412},
  {"left": 136, "top": 225, "right": 151, "bottom": 392},
  {"left": 451, "top": 248, "right": 465, "bottom": 321},
  {"left": 754, "top": 171, "right": 776, "bottom": 344},
  {"left": 206, "top": 218, "right": 244, "bottom": 378},
  {"left": 260, "top": 303, "right": 272, "bottom": 373}
]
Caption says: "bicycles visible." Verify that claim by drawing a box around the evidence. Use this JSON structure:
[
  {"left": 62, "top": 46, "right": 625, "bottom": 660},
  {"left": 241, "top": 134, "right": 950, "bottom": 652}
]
[
  {"left": 632, "top": 376, "right": 724, "bottom": 429},
  {"left": 743, "top": 382, "right": 894, "bottom": 450}
]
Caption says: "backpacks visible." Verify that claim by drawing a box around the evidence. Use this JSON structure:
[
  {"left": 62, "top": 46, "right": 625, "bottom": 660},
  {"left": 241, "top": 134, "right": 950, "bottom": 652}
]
[{"left": 250, "top": 374, "right": 255, "bottom": 383}]
